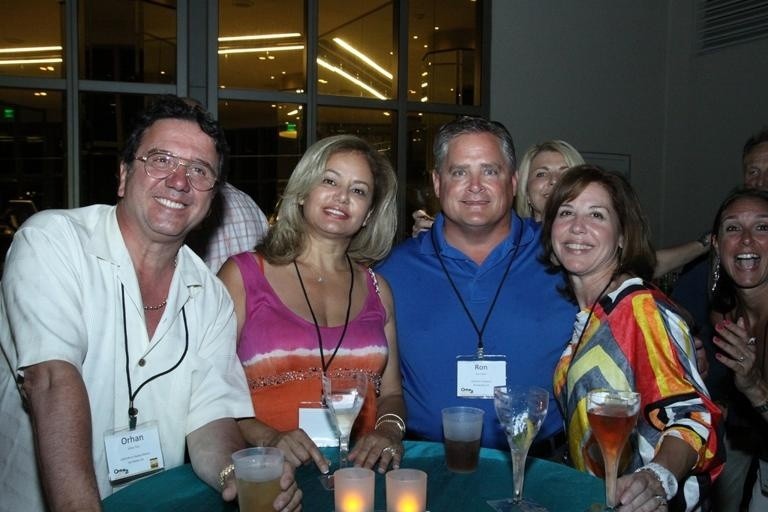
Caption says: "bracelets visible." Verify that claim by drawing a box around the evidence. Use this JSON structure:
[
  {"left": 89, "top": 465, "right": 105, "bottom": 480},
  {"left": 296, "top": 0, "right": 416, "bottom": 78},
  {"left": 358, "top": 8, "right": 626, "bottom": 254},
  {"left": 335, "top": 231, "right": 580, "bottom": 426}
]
[
  {"left": 695, "top": 233, "right": 711, "bottom": 252},
  {"left": 218, "top": 461, "right": 234, "bottom": 486},
  {"left": 374, "top": 412, "right": 407, "bottom": 434}
]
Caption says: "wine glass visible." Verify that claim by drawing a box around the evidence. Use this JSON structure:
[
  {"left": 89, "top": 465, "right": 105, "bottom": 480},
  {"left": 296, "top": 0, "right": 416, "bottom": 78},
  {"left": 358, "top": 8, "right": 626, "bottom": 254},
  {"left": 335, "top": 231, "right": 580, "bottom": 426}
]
[
  {"left": 316, "top": 367, "right": 370, "bottom": 492},
  {"left": 585, "top": 384, "right": 642, "bottom": 512},
  {"left": 484, "top": 382, "right": 549, "bottom": 512}
]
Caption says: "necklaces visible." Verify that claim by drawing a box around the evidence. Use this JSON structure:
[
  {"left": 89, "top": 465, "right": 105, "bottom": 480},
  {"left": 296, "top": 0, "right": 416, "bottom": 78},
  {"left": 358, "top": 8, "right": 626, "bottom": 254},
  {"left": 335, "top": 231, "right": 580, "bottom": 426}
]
[
  {"left": 143, "top": 255, "right": 177, "bottom": 310},
  {"left": 301, "top": 252, "right": 339, "bottom": 287}
]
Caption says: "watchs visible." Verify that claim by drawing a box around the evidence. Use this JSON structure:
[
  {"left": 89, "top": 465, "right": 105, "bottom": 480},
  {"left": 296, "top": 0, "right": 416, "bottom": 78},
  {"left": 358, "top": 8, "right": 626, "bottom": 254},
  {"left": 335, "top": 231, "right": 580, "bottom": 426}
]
[{"left": 634, "top": 461, "right": 679, "bottom": 501}]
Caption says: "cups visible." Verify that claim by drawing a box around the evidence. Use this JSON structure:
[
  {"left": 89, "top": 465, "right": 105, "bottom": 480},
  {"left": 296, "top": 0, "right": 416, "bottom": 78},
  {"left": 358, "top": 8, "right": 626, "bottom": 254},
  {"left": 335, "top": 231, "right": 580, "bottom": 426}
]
[
  {"left": 441, "top": 406, "right": 486, "bottom": 475},
  {"left": 332, "top": 468, "right": 376, "bottom": 512},
  {"left": 231, "top": 446, "right": 284, "bottom": 512},
  {"left": 384, "top": 468, "right": 427, "bottom": 512}
]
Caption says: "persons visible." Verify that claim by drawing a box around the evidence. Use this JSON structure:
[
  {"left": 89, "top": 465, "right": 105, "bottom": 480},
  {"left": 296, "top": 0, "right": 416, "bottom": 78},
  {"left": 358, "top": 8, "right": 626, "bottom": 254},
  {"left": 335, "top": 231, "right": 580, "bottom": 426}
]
[
  {"left": 538, "top": 161, "right": 729, "bottom": 511},
  {"left": 214, "top": 130, "right": 408, "bottom": 473},
  {"left": 690, "top": 189, "right": 767, "bottom": 512},
  {"left": 369, "top": 115, "right": 583, "bottom": 463},
  {"left": 184, "top": 173, "right": 275, "bottom": 279},
  {"left": 411, "top": 138, "right": 716, "bottom": 280},
  {"left": 3, "top": 93, "right": 304, "bottom": 512},
  {"left": 742, "top": 128, "right": 767, "bottom": 192}
]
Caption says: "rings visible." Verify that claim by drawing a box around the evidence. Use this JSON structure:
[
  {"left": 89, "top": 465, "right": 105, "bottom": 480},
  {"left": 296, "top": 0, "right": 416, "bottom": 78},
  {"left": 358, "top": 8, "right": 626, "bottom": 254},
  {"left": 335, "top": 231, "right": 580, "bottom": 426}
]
[
  {"left": 653, "top": 493, "right": 665, "bottom": 506},
  {"left": 746, "top": 333, "right": 755, "bottom": 347},
  {"left": 382, "top": 446, "right": 398, "bottom": 456},
  {"left": 737, "top": 350, "right": 746, "bottom": 362}
]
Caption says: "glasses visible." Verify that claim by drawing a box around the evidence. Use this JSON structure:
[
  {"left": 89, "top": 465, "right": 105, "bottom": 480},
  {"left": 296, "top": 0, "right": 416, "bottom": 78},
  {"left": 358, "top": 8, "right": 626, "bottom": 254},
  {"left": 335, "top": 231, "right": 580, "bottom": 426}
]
[{"left": 136, "top": 149, "right": 219, "bottom": 190}]
